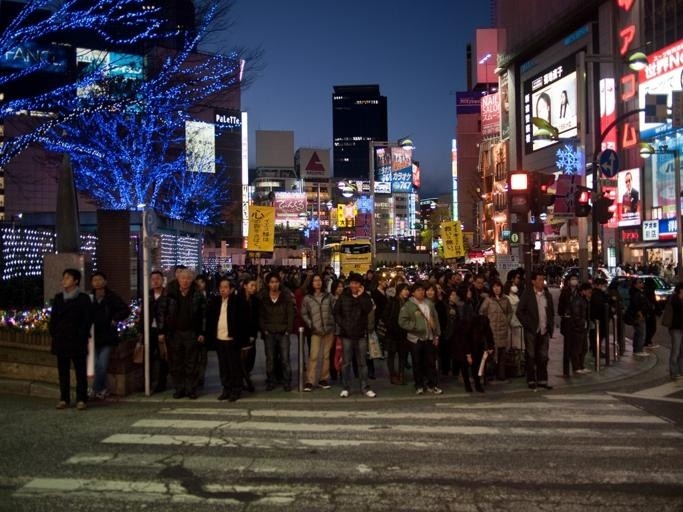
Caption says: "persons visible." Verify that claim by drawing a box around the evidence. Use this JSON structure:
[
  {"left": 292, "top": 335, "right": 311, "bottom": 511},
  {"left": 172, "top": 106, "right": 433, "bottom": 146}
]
[
  {"left": 536, "top": 91, "right": 552, "bottom": 129},
  {"left": 622, "top": 172, "right": 639, "bottom": 213},
  {"left": 48, "top": 258, "right": 683, "bottom": 410},
  {"left": 559, "top": 90, "right": 572, "bottom": 118}
]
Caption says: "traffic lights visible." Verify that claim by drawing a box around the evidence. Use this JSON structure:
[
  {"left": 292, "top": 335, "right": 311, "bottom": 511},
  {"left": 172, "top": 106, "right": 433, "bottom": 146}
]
[
  {"left": 531, "top": 170, "right": 558, "bottom": 214},
  {"left": 505, "top": 169, "right": 534, "bottom": 215},
  {"left": 573, "top": 188, "right": 591, "bottom": 218},
  {"left": 596, "top": 193, "right": 614, "bottom": 226}
]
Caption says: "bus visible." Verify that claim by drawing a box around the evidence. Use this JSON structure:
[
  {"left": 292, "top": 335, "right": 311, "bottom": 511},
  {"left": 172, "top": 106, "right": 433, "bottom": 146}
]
[{"left": 320, "top": 238, "right": 371, "bottom": 277}]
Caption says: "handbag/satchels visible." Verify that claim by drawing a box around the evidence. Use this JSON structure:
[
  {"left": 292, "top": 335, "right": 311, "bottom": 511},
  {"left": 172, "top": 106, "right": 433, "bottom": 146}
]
[
  {"left": 433, "top": 336, "right": 439, "bottom": 346},
  {"left": 134, "top": 342, "right": 144, "bottom": 363}
]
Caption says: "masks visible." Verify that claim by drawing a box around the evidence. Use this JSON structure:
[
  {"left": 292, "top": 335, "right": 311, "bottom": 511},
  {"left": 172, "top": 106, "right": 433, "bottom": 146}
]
[
  {"left": 511, "top": 286, "right": 519, "bottom": 293},
  {"left": 571, "top": 280, "right": 577, "bottom": 286}
]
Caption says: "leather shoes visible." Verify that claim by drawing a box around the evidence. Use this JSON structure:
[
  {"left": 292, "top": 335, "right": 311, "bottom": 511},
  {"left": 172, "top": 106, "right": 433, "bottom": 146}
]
[{"left": 528, "top": 383, "right": 553, "bottom": 390}]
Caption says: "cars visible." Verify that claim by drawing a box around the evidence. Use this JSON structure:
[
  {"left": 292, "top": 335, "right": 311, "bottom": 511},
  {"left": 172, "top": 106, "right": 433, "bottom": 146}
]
[
  {"left": 607, "top": 274, "right": 675, "bottom": 306},
  {"left": 376, "top": 234, "right": 414, "bottom": 244},
  {"left": 378, "top": 262, "right": 474, "bottom": 284}
]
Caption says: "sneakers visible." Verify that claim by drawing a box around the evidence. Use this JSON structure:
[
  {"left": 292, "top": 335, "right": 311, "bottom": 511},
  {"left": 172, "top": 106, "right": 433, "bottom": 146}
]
[
  {"left": 304, "top": 383, "right": 313, "bottom": 392},
  {"left": 77, "top": 402, "right": 86, "bottom": 410},
  {"left": 427, "top": 387, "right": 443, "bottom": 394},
  {"left": 416, "top": 388, "right": 424, "bottom": 396},
  {"left": 56, "top": 401, "right": 66, "bottom": 409},
  {"left": 632, "top": 344, "right": 660, "bottom": 357},
  {"left": 87, "top": 387, "right": 109, "bottom": 400},
  {"left": 318, "top": 380, "right": 331, "bottom": 389},
  {"left": 576, "top": 368, "right": 592, "bottom": 374},
  {"left": 340, "top": 390, "right": 349, "bottom": 397},
  {"left": 365, "top": 390, "right": 376, "bottom": 397}
]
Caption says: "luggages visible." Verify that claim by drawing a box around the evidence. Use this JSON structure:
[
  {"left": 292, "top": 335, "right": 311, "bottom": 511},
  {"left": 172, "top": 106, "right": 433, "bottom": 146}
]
[{"left": 507, "top": 326, "right": 526, "bottom": 377}]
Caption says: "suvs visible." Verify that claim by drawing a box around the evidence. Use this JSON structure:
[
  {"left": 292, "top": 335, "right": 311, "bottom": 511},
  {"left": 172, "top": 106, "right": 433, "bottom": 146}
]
[{"left": 558, "top": 265, "right": 629, "bottom": 293}]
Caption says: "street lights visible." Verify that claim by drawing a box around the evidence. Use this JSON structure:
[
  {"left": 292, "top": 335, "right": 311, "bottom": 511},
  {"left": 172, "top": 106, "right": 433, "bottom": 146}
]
[
  {"left": 298, "top": 182, "right": 344, "bottom": 268},
  {"left": 533, "top": 48, "right": 651, "bottom": 294},
  {"left": 342, "top": 137, "right": 414, "bottom": 272},
  {"left": 639, "top": 144, "right": 682, "bottom": 288}
]
